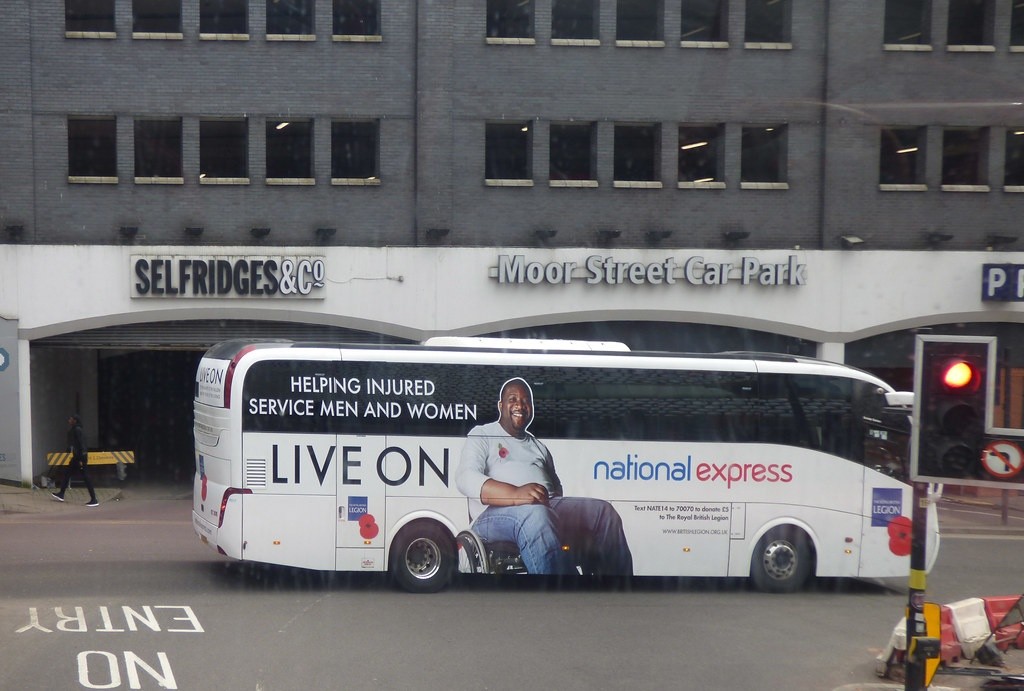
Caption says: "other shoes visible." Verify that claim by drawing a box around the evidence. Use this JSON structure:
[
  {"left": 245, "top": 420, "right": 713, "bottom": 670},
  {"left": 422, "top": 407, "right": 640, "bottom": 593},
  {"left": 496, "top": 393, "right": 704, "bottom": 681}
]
[
  {"left": 85, "top": 499, "right": 99, "bottom": 507},
  {"left": 52, "top": 492, "right": 64, "bottom": 501}
]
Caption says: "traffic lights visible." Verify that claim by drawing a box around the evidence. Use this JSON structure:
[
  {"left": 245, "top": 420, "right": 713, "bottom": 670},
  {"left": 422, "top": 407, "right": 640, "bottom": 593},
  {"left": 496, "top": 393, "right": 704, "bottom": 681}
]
[{"left": 907, "top": 334, "right": 998, "bottom": 487}]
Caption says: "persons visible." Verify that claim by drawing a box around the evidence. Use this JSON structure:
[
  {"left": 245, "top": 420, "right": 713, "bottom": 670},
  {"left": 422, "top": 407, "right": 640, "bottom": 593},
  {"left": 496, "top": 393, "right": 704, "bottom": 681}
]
[
  {"left": 454, "top": 377, "right": 634, "bottom": 575},
  {"left": 51, "top": 413, "right": 99, "bottom": 507}
]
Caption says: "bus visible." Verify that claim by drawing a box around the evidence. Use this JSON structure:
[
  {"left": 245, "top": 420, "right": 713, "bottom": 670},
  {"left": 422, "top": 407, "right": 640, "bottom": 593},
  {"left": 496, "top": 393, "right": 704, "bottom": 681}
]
[{"left": 190, "top": 335, "right": 944, "bottom": 594}]
